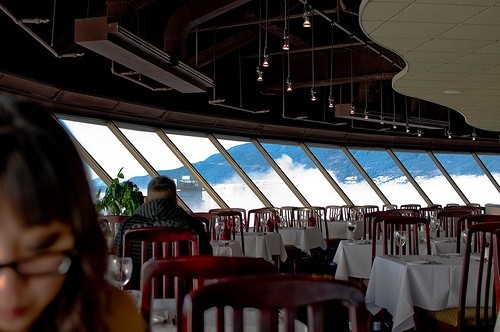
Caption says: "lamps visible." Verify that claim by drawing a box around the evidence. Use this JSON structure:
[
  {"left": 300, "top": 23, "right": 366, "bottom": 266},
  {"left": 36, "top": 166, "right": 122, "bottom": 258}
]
[
  {"left": 301, "top": 2, "right": 313, "bottom": 27},
  {"left": 283, "top": 0, "right": 290, "bottom": 50},
  {"left": 287, "top": 52, "right": 293, "bottom": 92},
  {"left": 328, "top": 23, "right": 421, "bottom": 136},
  {"left": 310, "top": 18, "right": 316, "bottom": 102},
  {"left": 447, "top": 108, "right": 451, "bottom": 138},
  {"left": 472, "top": 128, "right": 476, "bottom": 141},
  {"left": 257, "top": 16, "right": 263, "bottom": 81},
  {"left": 263, "top": 0, "right": 269, "bottom": 68}
]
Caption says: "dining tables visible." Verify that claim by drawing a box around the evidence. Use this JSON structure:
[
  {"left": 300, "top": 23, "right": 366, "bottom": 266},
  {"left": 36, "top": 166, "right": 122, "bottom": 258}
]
[
  {"left": 239, "top": 231, "right": 281, "bottom": 260},
  {"left": 211, "top": 240, "right": 240, "bottom": 257},
  {"left": 365, "top": 252, "right": 491, "bottom": 332},
  {"left": 332, "top": 236, "right": 462, "bottom": 281},
  {"left": 280, "top": 226, "right": 328, "bottom": 251},
  {"left": 323, "top": 219, "right": 369, "bottom": 239}
]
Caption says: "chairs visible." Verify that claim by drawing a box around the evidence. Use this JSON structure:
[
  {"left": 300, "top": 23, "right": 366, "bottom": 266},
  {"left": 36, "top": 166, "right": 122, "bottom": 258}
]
[{"left": 103, "top": 203, "right": 500, "bottom": 332}]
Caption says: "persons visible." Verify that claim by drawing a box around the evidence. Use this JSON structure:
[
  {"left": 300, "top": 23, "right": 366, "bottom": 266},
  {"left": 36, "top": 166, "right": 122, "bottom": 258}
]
[
  {"left": 113, "top": 176, "right": 213, "bottom": 300},
  {"left": 0, "top": 93, "right": 146, "bottom": 332}
]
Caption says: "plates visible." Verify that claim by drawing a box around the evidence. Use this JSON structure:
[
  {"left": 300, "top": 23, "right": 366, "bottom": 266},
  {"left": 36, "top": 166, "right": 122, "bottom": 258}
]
[{"left": 403, "top": 260, "right": 429, "bottom": 265}]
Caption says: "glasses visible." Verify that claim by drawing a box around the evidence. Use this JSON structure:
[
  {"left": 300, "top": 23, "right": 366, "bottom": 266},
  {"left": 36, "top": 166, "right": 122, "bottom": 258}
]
[{"left": 0, "top": 249, "right": 78, "bottom": 280}]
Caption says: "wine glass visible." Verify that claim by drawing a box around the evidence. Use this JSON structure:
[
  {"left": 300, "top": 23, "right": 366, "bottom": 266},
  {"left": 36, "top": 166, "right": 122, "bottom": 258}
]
[
  {"left": 235, "top": 218, "right": 241, "bottom": 233},
  {"left": 260, "top": 218, "right": 268, "bottom": 234},
  {"left": 394, "top": 231, "right": 407, "bottom": 259},
  {"left": 348, "top": 220, "right": 357, "bottom": 243},
  {"left": 214, "top": 222, "right": 225, "bottom": 245}
]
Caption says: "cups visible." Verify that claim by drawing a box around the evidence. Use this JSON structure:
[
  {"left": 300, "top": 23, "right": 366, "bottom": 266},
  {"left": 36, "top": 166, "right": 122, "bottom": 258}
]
[
  {"left": 104, "top": 255, "right": 133, "bottom": 291},
  {"left": 461, "top": 232, "right": 474, "bottom": 246}
]
